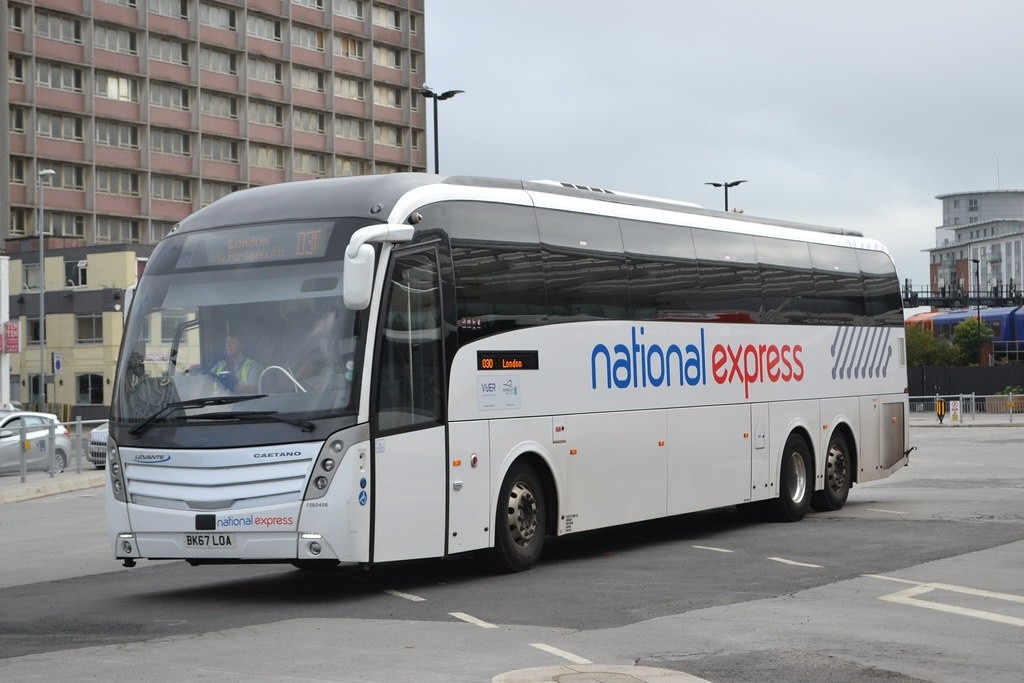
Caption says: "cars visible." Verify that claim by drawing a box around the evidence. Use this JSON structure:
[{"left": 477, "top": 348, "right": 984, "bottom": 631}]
[
  {"left": 87, "top": 422, "right": 108, "bottom": 469},
  {"left": 0, "top": 402, "right": 71, "bottom": 473}
]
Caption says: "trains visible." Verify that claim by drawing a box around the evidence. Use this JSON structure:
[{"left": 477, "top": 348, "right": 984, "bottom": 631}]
[{"left": 906, "top": 305, "right": 1024, "bottom": 360}]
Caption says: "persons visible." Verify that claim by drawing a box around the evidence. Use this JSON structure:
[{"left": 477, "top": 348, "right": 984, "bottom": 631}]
[{"left": 186, "top": 330, "right": 263, "bottom": 397}]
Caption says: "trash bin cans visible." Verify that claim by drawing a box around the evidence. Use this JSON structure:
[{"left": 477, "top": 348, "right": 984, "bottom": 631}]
[{"left": 950, "top": 401, "right": 960, "bottom": 423}]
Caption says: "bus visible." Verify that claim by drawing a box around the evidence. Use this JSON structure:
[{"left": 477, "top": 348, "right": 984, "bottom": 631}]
[{"left": 104, "top": 174, "right": 916, "bottom": 569}]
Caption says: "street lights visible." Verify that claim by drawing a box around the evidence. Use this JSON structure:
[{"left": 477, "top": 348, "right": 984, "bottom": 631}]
[
  {"left": 972, "top": 258, "right": 981, "bottom": 337},
  {"left": 418, "top": 87, "right": 465, "bottom": 174},
  {"left": 706, "top": 181, "right": 745, "bottom": 211},
  {"left": 35, "top": 168, "right": 57, "bottom": 413}
]
